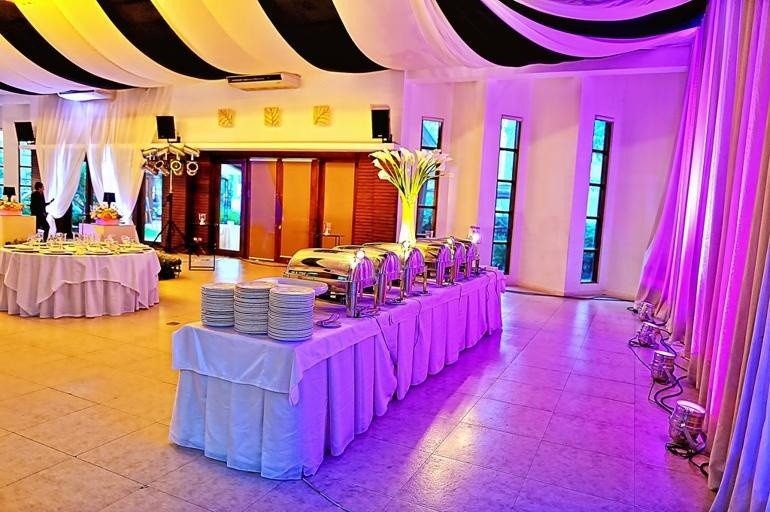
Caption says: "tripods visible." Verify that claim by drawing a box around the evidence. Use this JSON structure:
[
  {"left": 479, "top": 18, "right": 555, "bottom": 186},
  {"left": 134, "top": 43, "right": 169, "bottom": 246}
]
[{"left": 150, "top": 169, "right": 195, "bottom": 254}]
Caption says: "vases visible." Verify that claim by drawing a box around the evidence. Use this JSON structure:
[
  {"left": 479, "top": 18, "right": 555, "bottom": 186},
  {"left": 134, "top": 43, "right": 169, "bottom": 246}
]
[{"left": 400, "top": 195, "right": 419, "bottom": 251}]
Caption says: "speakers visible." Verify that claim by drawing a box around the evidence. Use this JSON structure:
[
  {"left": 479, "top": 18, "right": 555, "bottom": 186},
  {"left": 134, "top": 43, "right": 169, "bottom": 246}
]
[
  {"left": 371, "top": 108, "right": 390, "bottom": 139},
  {"left": 14, "top": 121, "right": 35, "bottom": 141},
  {"left": 156, "top": 115, "right": 176, "bottom": 139}
]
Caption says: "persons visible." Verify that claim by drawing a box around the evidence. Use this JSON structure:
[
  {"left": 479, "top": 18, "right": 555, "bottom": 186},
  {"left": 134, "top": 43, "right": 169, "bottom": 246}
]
[{"left": 29, "top": 182, "right": 55, "bottom": 242}]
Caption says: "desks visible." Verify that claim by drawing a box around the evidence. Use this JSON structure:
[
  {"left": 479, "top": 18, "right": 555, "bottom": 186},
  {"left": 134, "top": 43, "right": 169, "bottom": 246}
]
[
  {"left": 0, "top": 214, "right": 37, "bottom": 252},
  {"left": 172, "top": 260, "right": 506, "bottom": 483},
  {"left": 187, "top": 224, "right": 217, "bottom": 272},
  {"left": 314, "top": 234, "right": 343, "bottom": 251},
  {"left": 78, "top": 223, "right": 141, "bottom": 244}
]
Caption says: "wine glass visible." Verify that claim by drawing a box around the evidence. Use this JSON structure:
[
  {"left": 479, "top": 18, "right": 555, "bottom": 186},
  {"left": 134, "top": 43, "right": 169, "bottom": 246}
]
[{"left": 31, "top": 233, "right": 132, "bottom": 253}]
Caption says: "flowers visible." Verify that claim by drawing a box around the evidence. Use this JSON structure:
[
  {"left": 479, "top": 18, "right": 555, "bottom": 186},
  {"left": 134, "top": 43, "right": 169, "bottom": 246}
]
[
  {"left": 0, "top": 200, "right": 24, "bottom": 211},
  {"left": 89, "top": 206, "right": 123, "bottom": 220},
  {"left": 368, "top": 144, "right": 455, "bottom": 205}
]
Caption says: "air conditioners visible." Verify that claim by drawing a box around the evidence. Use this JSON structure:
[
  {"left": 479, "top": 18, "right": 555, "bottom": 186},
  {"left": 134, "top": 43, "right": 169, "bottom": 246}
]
[
  {"left": 55, "top": 88, "right": 116, "bottom": 102},
  {"left": 226, "top": 72, "right": 302, "bottom": 92}
]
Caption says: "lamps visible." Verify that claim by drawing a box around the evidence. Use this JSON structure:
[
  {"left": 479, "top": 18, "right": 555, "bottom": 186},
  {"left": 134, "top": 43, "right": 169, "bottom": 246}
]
[
  {"left": 3, "top": 187, "right": 16, "bottom": 202},
  {"left": 103, "top": 193, "right": 116, "bottom": 208},
  {"left": 140, "top": 146, "right": 201, "bottom": 177},
  {"left": 633, "top": 298, "right": 708, "bottom": 458}
]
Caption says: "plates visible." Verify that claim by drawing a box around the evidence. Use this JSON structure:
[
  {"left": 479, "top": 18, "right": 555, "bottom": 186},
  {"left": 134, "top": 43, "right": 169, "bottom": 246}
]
[
  {"left": 4, "top": 241, "right": 151, "bottom": 255},
  {"left": 315, "top": 290, "right": 431, "bottom": 328},
  {"left": 200, "top": 276, "right": 329, "bottom": 342}
]
